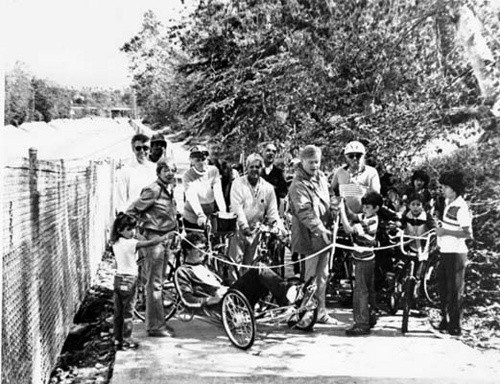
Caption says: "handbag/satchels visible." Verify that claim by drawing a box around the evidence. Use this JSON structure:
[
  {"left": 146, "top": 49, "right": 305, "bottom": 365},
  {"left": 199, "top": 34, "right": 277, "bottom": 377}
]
[{"left": 114, "top": 274, "right": 137, "bottom": 296}]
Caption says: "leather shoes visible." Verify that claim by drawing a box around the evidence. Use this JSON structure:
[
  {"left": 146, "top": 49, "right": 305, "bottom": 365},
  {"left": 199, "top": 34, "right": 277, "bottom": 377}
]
[{"left": 345, "top": 326, "right": 371, "bottom": 335}]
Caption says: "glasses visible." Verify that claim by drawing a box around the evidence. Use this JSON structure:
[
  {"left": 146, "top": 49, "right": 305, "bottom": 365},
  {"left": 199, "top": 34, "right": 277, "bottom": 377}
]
[
  {"left": 134, "top": 144, "right": 149, "bottom": 151},
  {"left": 194, "top": 246, "right": 207, "bottom": 251},
  {"left": 347, "top": 154, "right": 361, "bottom": 158}
]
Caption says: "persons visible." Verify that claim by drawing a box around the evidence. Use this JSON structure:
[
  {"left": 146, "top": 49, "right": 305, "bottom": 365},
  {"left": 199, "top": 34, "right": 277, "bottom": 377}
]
[
  {"left": 105, "top": 211, "right": 177, "bottom": 349},
  {"left": 228, "top": 151, "right": 286, "bottom": 287},
  {"left": 182, "top": 145, "right": 227, "bottom": 242},
  {"left": 287, "top": 144, "right": 339, "bottom": 326},
  {"left": 147, "top": 133, "right": 169, "bottom": 164},
  {"left": 115, "top": 134, "right": 159, "bottom": 213},
  {"left": 393, "top": 194, "right": 436, "bottom": 316},
  {"left": 330, "top": 141, "right": 382, "bottom": 215},
  {"left": 174, "top": 233, "right": 306, "bottom": 318},
  {"left": 336, "top": 191, "right": 382, "bottom": 337},
  {"left": 395, "top": 169, "right": 434, "bottom": 221},
  {"left": 427, "top": 171, "right": 473, "bottom": 340},
  {"left": 259, "top": 143, "right": 287, "bottom": 214},
  {"left": 126, "top": 157, "right": 180, "bottom": 338}
]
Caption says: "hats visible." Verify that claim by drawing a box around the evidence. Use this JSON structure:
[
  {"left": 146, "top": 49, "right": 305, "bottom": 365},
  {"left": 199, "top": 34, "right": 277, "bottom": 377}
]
[
  {"left": 344, "top": 140, "right": 366, "bottom": 153},
  {"left": 150, "top": 133, "right": 166, "bottom": 145}
]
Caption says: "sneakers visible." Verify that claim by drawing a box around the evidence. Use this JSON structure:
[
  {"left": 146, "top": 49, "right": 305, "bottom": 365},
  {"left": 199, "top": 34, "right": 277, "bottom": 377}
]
[
  {"left": 148, "top": 326, "right": 176, "bottom": 337},
  {"left": 316, "top": 313, "right": 338, "bottom": 325},
  {"left": 295, "top": 275, "right": 315, "bottom": 307},
  {"left": 122, "top": 341, "right": 138, "bottom": 349}
]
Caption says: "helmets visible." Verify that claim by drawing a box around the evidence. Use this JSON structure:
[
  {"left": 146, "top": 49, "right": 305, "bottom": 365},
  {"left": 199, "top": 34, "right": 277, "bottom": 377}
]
[{"left": 188, "top": 144, "right": 210, "bottom": 157}]
